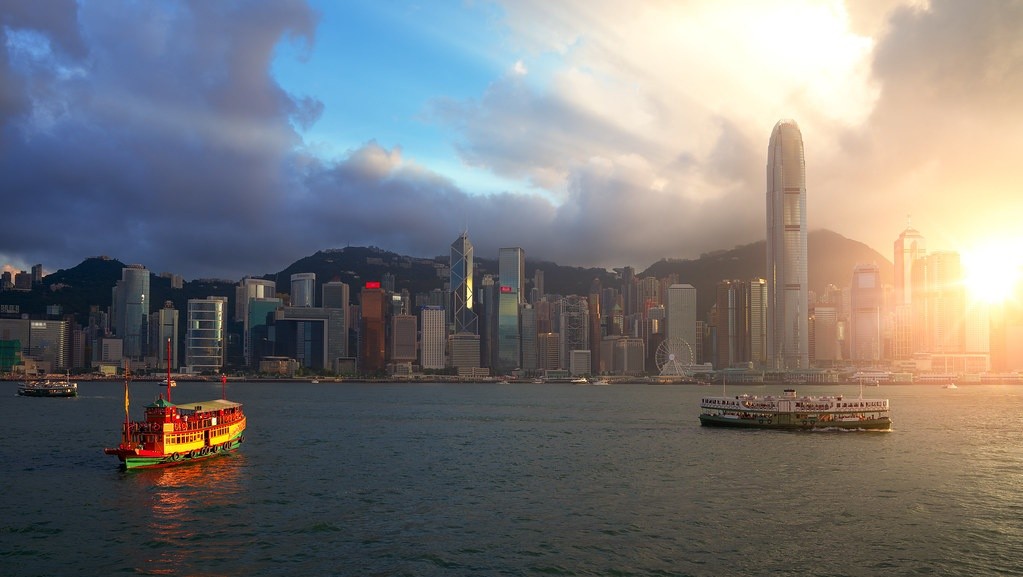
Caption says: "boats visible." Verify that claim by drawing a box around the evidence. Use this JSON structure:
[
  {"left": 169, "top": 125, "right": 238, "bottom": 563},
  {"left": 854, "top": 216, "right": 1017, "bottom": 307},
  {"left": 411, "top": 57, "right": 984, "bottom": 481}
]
[
  {"left": 103, "top": 337, "right": 248, "bottom": 470},
  {"left": 943, "top": 383, "right": 959, "bottom": 389},
  {"left": 16, "top": 369, "right": 79, "bottom": 398},
  {"left": 696, "top": 374, "right": 892, "bottom": 431}
]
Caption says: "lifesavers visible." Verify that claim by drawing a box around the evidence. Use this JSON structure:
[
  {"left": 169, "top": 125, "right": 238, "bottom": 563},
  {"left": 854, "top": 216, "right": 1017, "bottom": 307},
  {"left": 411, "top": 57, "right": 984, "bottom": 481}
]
[
  {"left": 181, "top": 423, "right": 187, "bottom": 429},
  {"left": 190, "top": 450, "right": 196, "bottom": 458},
  {"left": 174, "top": 452, "right": 180, "bottom": 461},
  {"left": 810, "top": 420, "right": 815, "bottom": 425},
  {"left": 230, "top": 441, "right": 233, "bottom": 449},
  {"left": 214, "top": 446, "right": 219, "bottom": 452},
  {"left": 241, "top": 435, "right": 246, "bottom": 443},
  {"left": 174, "top": 423, "right": 179, "bottom": 431},
  {"left": 224, "top": 443, "right": 229, "bottom": 449},
  {"left": 206, "top": 446, "right": 210, "bottom": 454},
  {"left": 803, "top": 421, "right": 807, "bottom": 425},
  {"left": 767, "top": 419, "right": 772, "bottom": 424},
  {"left": 201, "top": 448, "right": 206, "bottom": 455},
  {"left": 153, "top": 424, "right": 160, "bottom": 430},
  {"left": 759, "top": 419, "right": 764, "bottom": 424}
]
